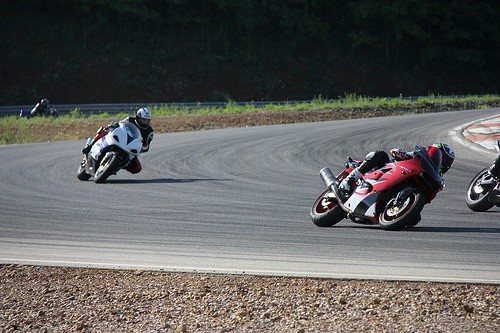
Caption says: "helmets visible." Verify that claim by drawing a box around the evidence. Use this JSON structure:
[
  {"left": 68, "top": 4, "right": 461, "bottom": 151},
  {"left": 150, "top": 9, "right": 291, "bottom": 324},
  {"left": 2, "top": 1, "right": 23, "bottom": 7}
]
[
  {"left": 429, "top": 142, "right": 454, "bottom": 176},
  {"left": 134, "top": 108, "right": 151, "bottom": 129},
  {"left": 41, "top": 99, "right": 48, "bottom": 106}
]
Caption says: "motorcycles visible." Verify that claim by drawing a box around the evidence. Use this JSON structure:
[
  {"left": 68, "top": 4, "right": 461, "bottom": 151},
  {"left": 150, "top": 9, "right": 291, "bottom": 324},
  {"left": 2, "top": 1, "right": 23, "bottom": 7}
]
[
  {"left": 310, "top": 144, "right": 446, "bottom": 230},
  {"left": 20, "top": 104, "right": 60, "bottom": 117},
  {"left": 464, "top": 140, "right": 500, "bottom": 213},
  {"left": 77, "top": 120, "right": 144, "bottom": 184}
]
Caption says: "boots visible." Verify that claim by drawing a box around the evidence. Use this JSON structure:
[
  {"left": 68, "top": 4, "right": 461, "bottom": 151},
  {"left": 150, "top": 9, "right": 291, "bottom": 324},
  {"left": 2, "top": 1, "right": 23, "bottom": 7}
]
[
  {"left": 488, "top": 154, "right": 500, "bottom": 176},
  {"left": 82, "top": 137, "right": 97, "bottom": 154},
  {"left": 338, "top": 167, "right": 363, "bottom": 201}
]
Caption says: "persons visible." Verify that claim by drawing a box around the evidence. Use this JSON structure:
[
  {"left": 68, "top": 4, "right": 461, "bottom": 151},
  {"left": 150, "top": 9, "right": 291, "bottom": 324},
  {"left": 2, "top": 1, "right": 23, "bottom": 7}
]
[
  {"left": 81, "top": 108, "right": 154, "bottom": 175},
  {"left": 30, "top": 99, "right": 53, "bottom": 118},
  {"left": 340, "top": 142, "right": 455, "bottom": 226}
]
